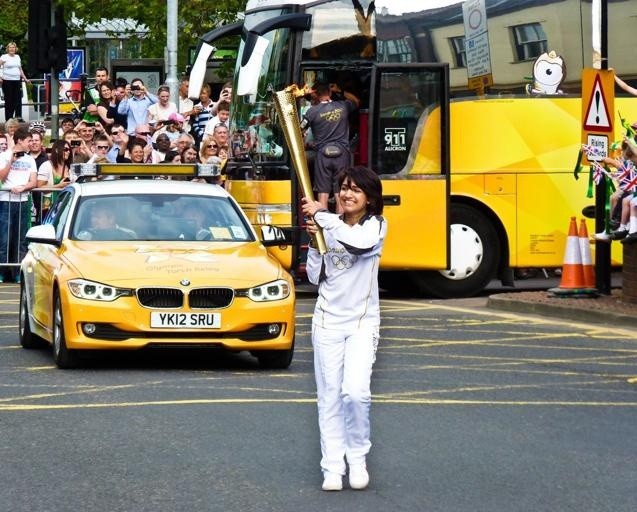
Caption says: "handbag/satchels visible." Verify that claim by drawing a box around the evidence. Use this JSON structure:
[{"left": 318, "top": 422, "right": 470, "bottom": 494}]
[{"left": 323, "top": 145, "right": 342, "bottom": 158}]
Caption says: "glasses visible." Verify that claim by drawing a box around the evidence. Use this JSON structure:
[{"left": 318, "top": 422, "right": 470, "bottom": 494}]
[
  {"left": 96, "top": 146, "right": 108, "bottom": 150},
  {"left": 207, "top": 145, "right": 217, "bottom": 148}
]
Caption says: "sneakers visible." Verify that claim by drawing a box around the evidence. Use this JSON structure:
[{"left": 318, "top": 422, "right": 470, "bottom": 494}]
[
  {"left": 321, "top": 472, "right": 343, "bottom": 492},
  {"left": 591, "top": 228, "right": 637, "bottom": 243},
  {"left": 349, "top": 462, "right": 370, "bottom": 490}
]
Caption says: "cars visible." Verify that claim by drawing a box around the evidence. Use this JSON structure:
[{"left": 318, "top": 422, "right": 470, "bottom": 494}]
[{"left": 18, "top": 163, "right": 296, "bottom": 369}]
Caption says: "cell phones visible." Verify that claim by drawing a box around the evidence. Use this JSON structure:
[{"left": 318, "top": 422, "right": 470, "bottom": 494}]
[
  {"left": 64, "top": 177, "right": 70, "bottom": 182},
  {"left": 86, "top": 123, "right": 95, "bottom": 127},
  {"left": 17, "top": 152, "right": 24, "bottom": 156},
  {"left": 162, "top": 121, "right": 172, "bottom": 125},
  {"left": 131, "top": 86, "right": 140, "bottom": 90},
  {"left": 223, "top": 90, "right": 228, "bottom": 96},
  {"left": 71, "top": 141, "right": 80, "bottom": 145}
]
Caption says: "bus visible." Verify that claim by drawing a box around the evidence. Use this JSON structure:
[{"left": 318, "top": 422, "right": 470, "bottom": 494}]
[{"left": 187, "top": 0, "right": 637, "bottom": 299}]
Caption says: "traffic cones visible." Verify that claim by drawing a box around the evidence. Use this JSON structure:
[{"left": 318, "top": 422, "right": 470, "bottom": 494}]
[{"left": 548, "top": 213, "right": 599, "bottom": 298}]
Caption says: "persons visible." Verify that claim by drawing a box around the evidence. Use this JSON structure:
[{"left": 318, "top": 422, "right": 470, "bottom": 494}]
[
  {"left": 233, "top": 108, "right": 280, "bottom": 164},
  {"left": 298, "top": 80, "right": 377, "bottom": 215},
  {"left": 588, "top": 67, "right": 637, "bottom": 245},
  {"left": 307, "top": 166, "right": 386, "bottom": 490},
  {"left": 0, "top": 41, "right": 33, "bottom": 124},
  {"left": 0, "top": 67, "right": 232, "bottom": 285}
]
